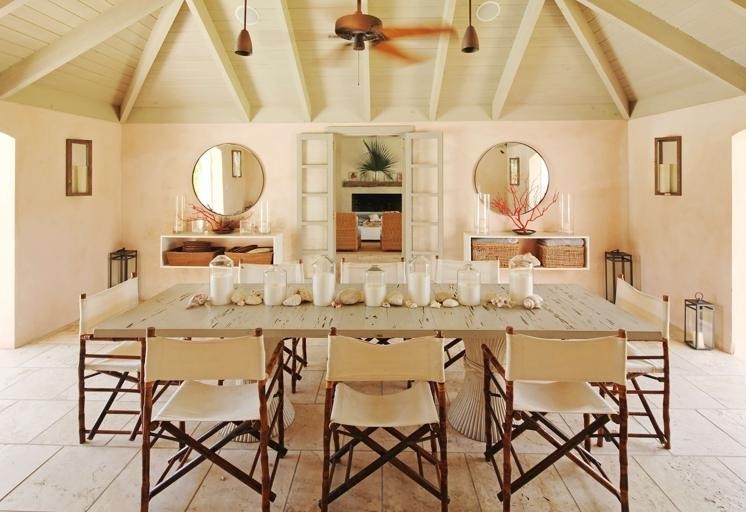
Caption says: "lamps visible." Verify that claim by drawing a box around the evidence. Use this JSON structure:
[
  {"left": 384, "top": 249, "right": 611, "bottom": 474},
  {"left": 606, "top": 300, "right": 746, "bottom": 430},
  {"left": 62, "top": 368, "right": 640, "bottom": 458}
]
[
  {"left": 461, "top": 0, "right": 479, "bottom": 52},
  {"left": 234, "top": 0, "right": 252, "bottom": 55}
]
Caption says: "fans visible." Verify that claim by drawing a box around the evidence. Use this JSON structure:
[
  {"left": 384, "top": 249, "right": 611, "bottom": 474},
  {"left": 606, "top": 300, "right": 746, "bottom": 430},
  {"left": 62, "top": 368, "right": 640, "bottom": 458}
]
[{"left": 335, "top": 0, "right": 455, "bottom": 64}]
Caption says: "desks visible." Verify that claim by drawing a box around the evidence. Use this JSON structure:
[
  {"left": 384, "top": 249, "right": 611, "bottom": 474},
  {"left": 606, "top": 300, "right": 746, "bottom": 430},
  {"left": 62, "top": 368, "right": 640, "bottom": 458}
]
[{"left": 92, "top": 281, "right": 664, "bottom": 445}]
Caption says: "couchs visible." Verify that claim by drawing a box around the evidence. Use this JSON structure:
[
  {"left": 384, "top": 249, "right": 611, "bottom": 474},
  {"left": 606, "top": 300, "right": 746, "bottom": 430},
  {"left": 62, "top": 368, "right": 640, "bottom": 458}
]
[{"left": 356, "top": 211, "right": 400, "bottom": 241}]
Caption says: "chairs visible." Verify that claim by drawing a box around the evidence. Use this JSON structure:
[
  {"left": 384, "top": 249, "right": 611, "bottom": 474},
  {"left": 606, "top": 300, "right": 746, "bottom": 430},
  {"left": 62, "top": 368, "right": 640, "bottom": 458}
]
[
  {"left": 337, "top": 212, "right": 361, "bottom": 252},
  {"left": 231, "top": 256, "right": 501, "bottom": 394},
  {"left": 380, "top": 212, "right": 402, "bottom": 251},
  {"left": 76, "top": 271, "right": 177, "bottom": 447},
  {"left": 317, "top": 326, "right": 449, "bottom": 512},
  {"left": 583, "top": 274, "right": 670, "bottom": 453},
  {"left": 480, "top": 325, "right": 631, "bottom": 512},
  {"left": 139, "top": 327, "right": 289, "bottom": 512}
]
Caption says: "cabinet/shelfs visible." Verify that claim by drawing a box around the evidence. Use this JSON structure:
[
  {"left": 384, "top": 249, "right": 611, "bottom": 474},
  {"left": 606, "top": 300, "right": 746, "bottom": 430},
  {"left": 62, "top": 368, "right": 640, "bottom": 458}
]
[
  {"left": 159, "top": 234, "right": 282, "bottom": 269},
  {"left": 604, "top": 252, "right": 633, "bottom": 304},
  {"left": 463, "top": 232, "right": 590, "bottom": 272}
]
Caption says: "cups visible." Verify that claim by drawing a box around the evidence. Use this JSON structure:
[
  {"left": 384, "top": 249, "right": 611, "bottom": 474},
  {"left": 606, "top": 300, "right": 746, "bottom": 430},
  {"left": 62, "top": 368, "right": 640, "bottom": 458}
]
[
  {"left": 559, "top": 191, "right": 573, "bottom": 235},
  {"left": 474, "top": 194, "right": 490, "bottom": 234},
  {"left": 171, "top": 193, "right": 272, "bottom": 237}
]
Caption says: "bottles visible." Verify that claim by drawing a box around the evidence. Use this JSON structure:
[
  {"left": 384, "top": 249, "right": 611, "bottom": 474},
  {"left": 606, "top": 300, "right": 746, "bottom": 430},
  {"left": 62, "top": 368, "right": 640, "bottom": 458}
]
[
  {"left": 309, "top": 248, "right": 338, "bottom": 309},
  {"left": 456, "top": 260, "right": 482, "bottom": 307},
  {"left": 208, "top": 254, "right": 233, "bottom": 306},
  {"left": 405, "top": 251, "right": 431, "bottom": 309},
  {"left": 261, "top": 264, "right": 289, "bottom": 307},
  {"left": 508, "top": 260, "right": 535, "bottom": 306},
  {"left": 363, "top": 263, "right": 389, "bottom": 309}
]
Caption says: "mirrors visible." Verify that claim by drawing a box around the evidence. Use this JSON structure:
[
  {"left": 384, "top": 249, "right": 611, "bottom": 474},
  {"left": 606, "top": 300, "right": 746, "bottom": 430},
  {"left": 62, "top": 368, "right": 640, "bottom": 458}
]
[
  {"left": 192, "top": 143, "right": 265, "bottom": 216},
  {"left": 474, "top": 141, "right": 550, "bottom": 216}
]
